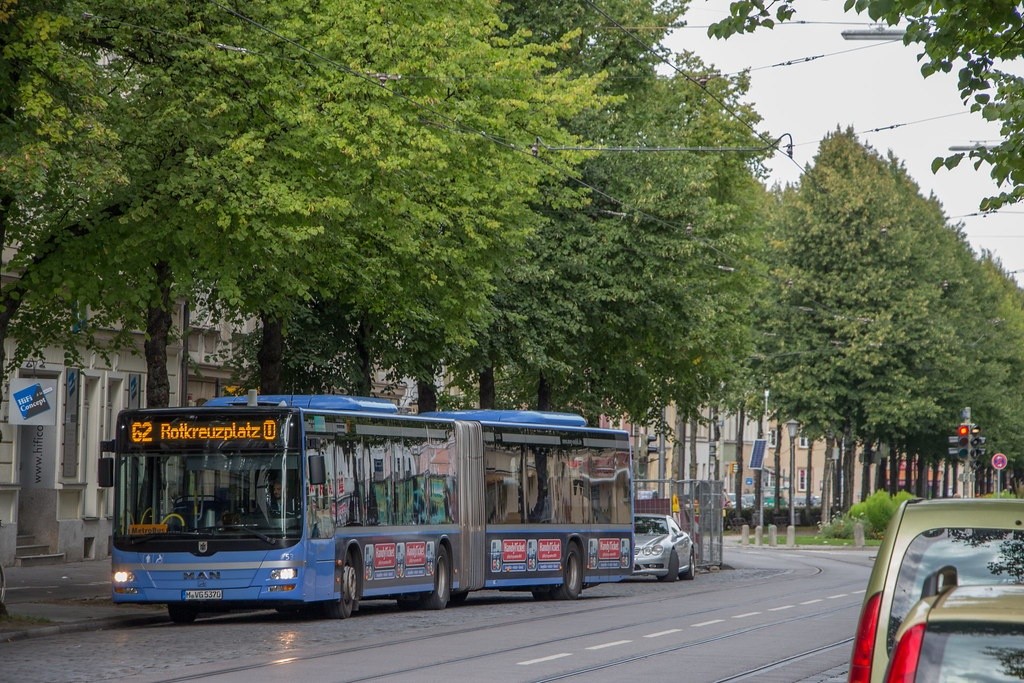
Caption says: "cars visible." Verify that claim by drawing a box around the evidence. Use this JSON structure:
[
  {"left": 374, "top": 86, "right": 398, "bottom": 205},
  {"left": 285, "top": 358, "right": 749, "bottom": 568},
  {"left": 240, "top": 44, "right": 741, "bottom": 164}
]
[
  {"left": 628, "top": 512, "right": 698, "bottom": 582},
  {"left": 881, "top": 563, "right": 1024, "bottom": 683}
]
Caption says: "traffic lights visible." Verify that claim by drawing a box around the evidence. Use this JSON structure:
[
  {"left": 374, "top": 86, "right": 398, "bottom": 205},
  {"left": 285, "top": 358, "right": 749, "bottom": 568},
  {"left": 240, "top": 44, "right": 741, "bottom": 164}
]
[
  {"left": 969, "top": 423, "right": 982, "bottom": 459},
  {"left": 957, "top": 424, "right": 969, "bottom": 459}
]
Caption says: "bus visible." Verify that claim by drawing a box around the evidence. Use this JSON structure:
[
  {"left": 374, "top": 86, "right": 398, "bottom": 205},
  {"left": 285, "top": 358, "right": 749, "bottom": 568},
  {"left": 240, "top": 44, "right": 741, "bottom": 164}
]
[{"left": 95, "top": 394, "right": 639, "bottom": 625}]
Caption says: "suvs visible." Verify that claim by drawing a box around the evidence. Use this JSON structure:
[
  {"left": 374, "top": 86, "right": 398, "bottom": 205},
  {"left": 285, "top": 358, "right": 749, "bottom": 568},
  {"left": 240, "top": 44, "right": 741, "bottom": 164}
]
[{"left": 847, "top": 494, "right": 1023, "bottom": 683}]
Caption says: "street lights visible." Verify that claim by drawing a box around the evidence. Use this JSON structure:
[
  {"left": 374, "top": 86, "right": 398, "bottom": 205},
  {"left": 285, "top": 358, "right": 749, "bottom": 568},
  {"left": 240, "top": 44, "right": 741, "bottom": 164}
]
[{"left": 785, "top": 417, "right": 800, "bottom": 547}]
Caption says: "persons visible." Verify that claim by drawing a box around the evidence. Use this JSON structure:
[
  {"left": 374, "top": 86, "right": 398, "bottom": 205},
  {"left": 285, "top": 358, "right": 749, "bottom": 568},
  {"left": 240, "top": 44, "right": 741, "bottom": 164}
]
[{"left": 269, "top": 480, "right": 295, "bottom": 518}]
